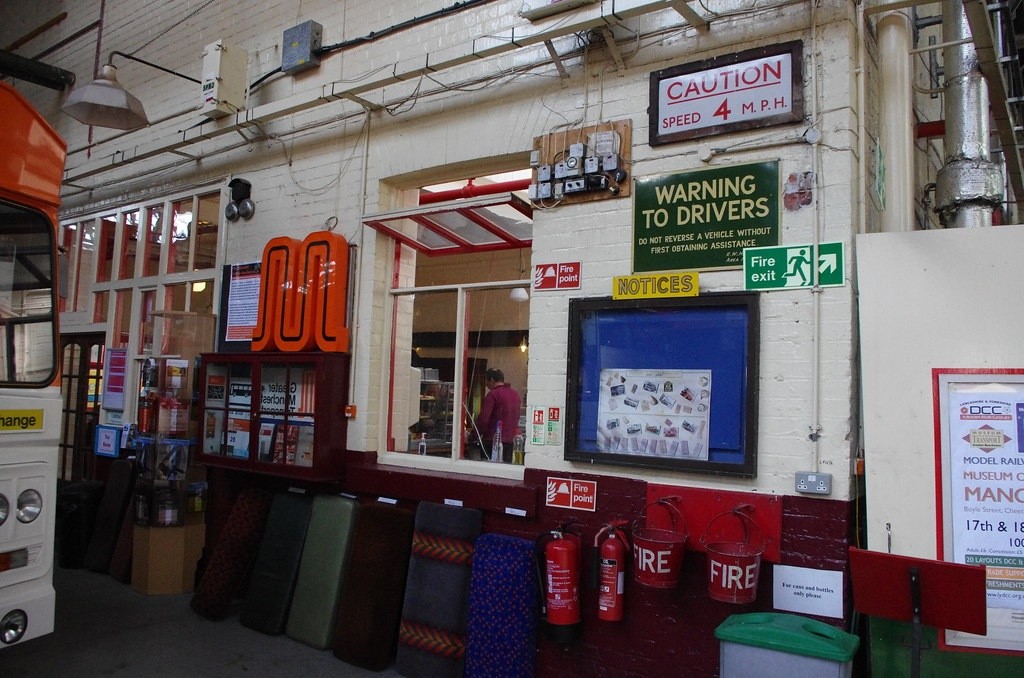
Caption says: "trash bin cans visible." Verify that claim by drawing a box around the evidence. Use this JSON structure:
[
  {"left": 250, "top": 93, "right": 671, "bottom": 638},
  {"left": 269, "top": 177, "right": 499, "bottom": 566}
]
[{"left": 714, "top": 611, "right": 860, "bottom": 678}]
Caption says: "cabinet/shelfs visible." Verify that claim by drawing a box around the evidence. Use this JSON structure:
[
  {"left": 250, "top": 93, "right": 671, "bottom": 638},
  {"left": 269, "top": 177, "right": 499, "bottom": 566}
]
[
  {"left": 193, "top": 351, "right": 350, "bottom": 485},
  {"left": 408, "top": 382, "right": 469, "bottom": 454},
  {"left": 132, "top": 309, "right": 216, "bottom": 595}
]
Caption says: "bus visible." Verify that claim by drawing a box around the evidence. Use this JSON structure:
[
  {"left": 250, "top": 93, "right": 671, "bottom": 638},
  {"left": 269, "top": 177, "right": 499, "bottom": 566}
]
[{"left": 0, "top": 83, "right": 67, "bottom": 649}]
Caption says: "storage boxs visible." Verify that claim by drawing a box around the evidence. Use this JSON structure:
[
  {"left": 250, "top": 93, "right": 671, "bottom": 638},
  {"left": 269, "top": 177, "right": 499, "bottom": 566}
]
[{"left": 135, "top": 438, "right": 189, "bottom": 481}]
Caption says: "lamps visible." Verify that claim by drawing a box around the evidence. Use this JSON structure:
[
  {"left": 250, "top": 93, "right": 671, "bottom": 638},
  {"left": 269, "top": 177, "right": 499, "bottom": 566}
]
[
  {"left": 510, "top": 248, "right": 529, "bottom": 301},
  {"left": 60, "top": 51, "right": 202, "bottom": 131},
  {"left": 518, "top": 336, "right": 528, "bottom": 352}
]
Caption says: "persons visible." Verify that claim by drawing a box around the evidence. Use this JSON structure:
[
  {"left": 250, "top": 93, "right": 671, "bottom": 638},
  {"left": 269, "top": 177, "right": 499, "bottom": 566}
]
[{"left": 467, "top": 367, "right": 522, "bottom": 466}]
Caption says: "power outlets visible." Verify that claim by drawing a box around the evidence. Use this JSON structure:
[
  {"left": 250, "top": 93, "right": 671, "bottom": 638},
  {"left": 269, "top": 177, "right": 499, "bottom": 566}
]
[{"left": 795, "top": 471, "right": 832, "bottom": 495}]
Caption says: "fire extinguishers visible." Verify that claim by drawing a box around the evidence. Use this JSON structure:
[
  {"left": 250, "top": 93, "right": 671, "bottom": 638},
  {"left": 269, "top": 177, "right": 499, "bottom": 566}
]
[
  {"left": 592, "top": 518, "right": 629, "bottom": 621},
  {"left": 533, "top": 520, "right": 586, "bottom": 626}
]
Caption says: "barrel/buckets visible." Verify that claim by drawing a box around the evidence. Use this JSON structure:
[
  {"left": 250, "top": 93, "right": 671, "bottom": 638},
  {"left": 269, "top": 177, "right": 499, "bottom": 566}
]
[
  {"left": 633, "top": 499, "right": 687, "bottom": 590},
  {"left": 703, "top": 510, "right": 768, "bottom": 604}
]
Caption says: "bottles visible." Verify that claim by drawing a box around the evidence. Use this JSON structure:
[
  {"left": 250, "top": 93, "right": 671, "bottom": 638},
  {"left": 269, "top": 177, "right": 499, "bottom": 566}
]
[
  {"left": 491, "top": 421, "right": 504, "bottom": 463},
  {"left": 512, "top": 428, "right": 525, "bottom": 465}
]
